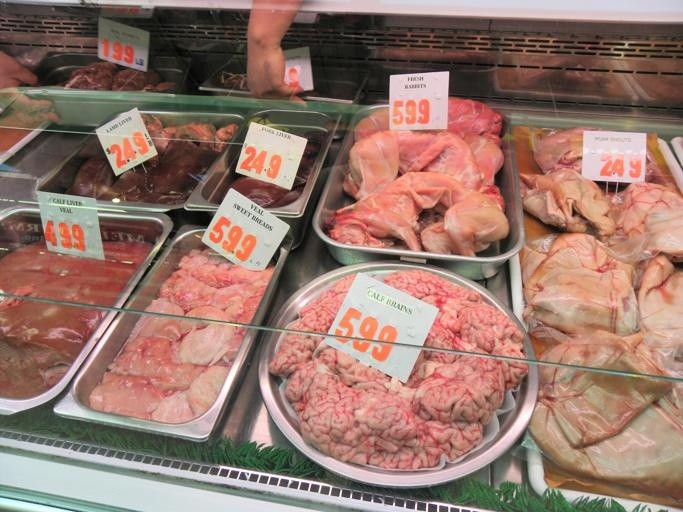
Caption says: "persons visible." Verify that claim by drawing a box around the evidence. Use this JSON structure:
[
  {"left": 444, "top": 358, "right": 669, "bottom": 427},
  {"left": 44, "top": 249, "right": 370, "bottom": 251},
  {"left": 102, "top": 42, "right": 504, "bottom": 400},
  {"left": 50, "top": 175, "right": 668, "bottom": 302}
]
[
  {"left": 1, "top": 51, "right": 59, "bottom": 128},
  {"left": 245, "top": 1, "right": 308, "bottom": 111}
]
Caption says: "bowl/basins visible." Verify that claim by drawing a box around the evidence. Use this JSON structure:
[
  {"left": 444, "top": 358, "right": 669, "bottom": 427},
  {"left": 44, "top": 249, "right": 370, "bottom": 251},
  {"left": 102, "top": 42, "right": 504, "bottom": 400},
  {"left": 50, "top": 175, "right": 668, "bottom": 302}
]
[{"left": 256, "top": 260, "right": 543, "bottom": 489}]
